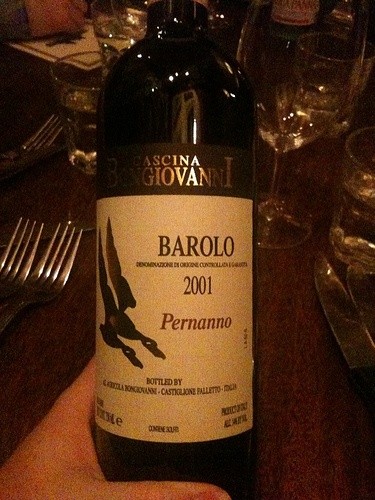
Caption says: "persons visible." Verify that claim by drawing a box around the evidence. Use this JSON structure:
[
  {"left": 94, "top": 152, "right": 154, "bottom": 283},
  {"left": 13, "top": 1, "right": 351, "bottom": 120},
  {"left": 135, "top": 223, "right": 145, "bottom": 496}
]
[
  {"left": 0, "top": 0, "right": 87, "bottom": 40},
  {"left": 0, "top": 350, "right": 233, "bottom": 500}
]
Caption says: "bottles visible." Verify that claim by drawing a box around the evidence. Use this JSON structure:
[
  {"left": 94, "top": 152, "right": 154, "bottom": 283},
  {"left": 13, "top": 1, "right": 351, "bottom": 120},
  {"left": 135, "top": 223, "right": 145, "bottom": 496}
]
[
  {"left": 95, "top": 0, "right": 252, "bottom": 500},
  {"left": 262, "top": 0, "right": 324, "bottom": 43}
]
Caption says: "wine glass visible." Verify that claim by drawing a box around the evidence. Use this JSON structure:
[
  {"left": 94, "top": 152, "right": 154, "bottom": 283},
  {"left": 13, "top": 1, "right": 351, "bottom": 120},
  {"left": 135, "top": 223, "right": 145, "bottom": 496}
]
[{"left": 236, "top": 0, "right": 369, "bottom": 248}]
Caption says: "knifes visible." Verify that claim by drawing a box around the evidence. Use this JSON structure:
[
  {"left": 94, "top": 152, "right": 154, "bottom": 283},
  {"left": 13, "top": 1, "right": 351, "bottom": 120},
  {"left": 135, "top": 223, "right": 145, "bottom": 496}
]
[
  {"left": 312, "top": 251, "right": 375, "bottom": 427},
  {"left": 0, "top": 144, "right": 67, "bottom": 182}
]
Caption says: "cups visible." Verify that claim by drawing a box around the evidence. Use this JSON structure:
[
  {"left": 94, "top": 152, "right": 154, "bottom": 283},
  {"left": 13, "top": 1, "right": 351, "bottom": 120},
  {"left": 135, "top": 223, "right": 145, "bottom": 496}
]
[
  {"left": 91, "top": 0, "right": 149, "bottom": 68},
  {"left": 50, "top": 50, "right": 122, "bottom": 174},
  {"left": 328, "top": 127, "right": 375, "bottom": 274},
  {"left": 296, "top": 32, "right": 375, "bottom": 137}
]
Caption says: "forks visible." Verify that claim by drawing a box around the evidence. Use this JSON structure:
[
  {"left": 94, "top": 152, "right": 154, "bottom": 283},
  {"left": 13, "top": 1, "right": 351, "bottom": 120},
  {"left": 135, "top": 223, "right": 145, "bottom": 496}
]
[
  {"left": 0, "top": 222, "right": 84, "bottom": 333},
  {"left": 0, "top": 115, "right": 66, "bottom": 169},
  {"left": 0, "top": 217, "right": 44, "bottom": 301}
]
